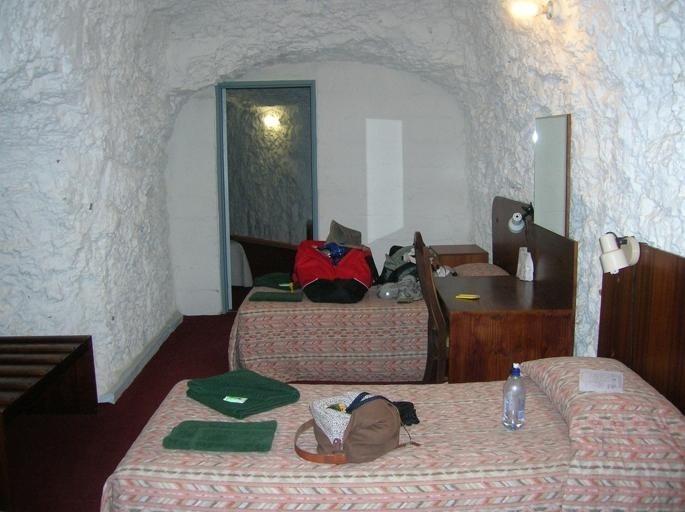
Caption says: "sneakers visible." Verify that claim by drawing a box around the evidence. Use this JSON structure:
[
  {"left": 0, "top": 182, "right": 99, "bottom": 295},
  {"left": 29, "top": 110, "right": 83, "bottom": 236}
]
[
  {"left": 378, "top": 275, "right": 416, "bottom": 298},
  {"left": 398, "top": 282, "right": 424, "bottom": 302}
]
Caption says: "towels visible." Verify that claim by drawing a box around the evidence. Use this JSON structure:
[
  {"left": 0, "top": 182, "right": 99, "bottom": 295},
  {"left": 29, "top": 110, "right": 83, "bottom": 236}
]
[
  {"left": 162, "top": 420, "right": 277, "bottom": 452},
  {"left": 186, "top": 368, "right": 300, "bottom": 418},
  {"left": 249, "top": 291, "right": 304, "bottom": 302}
]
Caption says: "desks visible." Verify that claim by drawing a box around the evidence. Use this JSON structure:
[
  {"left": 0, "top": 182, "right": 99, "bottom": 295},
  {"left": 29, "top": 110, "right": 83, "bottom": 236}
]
[
  {"left": 432, "top": 225, "right": 578, "bottom": 383},
  {"left": 0, "top": 334, "right": 100, "bottom": 512}
]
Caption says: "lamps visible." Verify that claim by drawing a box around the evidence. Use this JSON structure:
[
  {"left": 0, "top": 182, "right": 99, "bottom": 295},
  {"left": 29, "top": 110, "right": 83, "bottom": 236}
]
[
  {"left": 507, "top": 201, "right": 534, "bottom": 234},
  {"left": 597, "top": 232, "right": 641, "bottom": 276}
]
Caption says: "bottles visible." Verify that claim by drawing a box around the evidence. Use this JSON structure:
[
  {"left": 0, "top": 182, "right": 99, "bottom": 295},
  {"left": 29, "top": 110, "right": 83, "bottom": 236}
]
[
  {"left": 503, "top": 363, "right": 526, "bottom": 430},
  {"left": 315, "top": 242, "right": 344, "bottom": 260},
  {"left": 518, "top": 246, "right": 535, "bottom": 281}
]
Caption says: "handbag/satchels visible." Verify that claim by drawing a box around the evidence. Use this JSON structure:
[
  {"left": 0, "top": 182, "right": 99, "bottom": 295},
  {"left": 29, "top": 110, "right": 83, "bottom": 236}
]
[
  {"left": 294, "top": 241, "right": 379, "bottom": 303},
  {"left": 294, "top": 392, "right": 419, "bottom": 465}
]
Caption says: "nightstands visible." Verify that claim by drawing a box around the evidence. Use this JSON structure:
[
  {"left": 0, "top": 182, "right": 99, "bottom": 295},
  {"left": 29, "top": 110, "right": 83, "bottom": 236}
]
[{"left": 428, "top": 245, "right": 489, "bottom": 267}]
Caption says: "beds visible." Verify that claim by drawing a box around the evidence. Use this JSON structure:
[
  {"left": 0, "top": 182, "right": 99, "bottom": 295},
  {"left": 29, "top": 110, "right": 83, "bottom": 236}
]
[
  {"left": 228, "top": 196, "right": 535, "bottom": 383},
  {"left": 101, "top": 237, "right": 684, "bottom": 512}
]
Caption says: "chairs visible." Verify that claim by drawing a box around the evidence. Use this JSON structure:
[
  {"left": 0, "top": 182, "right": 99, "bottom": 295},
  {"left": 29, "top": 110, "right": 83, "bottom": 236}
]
[{"left": 413, "top": 231, "right": 450, "bottom": 383}]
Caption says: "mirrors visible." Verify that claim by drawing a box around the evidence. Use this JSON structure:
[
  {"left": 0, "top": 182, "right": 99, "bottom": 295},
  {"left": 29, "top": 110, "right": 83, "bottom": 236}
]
[{"left": 534, "top": 113, "right": 571, "bottom": 238}]
